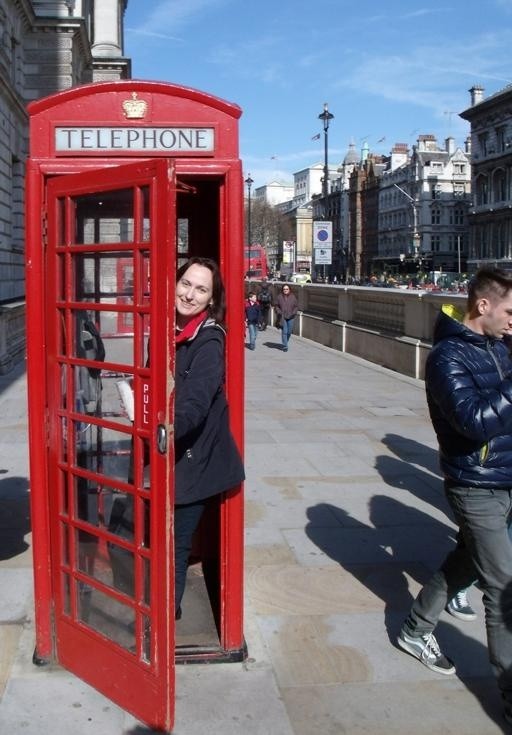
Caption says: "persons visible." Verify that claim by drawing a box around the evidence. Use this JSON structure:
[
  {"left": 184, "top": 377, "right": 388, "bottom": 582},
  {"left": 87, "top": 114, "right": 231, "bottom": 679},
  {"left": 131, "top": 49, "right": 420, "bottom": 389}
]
[
  {"left": 443, "top": 327, "right": 512, "bottom": 621},
  {"left": 394, "top": 262, "right": 512, "bottom": 724},
  {"left": 266, "top": 259, "right": 475, "bottom": 295},
  {"left": 275, "top": 283, "right": 298, "bottom": 353},
  {"left": 244, "top": 291, "right": 262, "bottom": 350},
  {"left": 254, "top": 277, "right": 274, "bottom": 330},
  {"left": 102, "top": 254, "right": 246, "bottom": 669}
]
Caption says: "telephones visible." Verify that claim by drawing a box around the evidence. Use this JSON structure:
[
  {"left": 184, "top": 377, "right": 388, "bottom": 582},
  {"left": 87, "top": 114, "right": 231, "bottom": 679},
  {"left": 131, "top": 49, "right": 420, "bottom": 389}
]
[{"left": 80, "top": 320, "right": 106, "bottom": 378}]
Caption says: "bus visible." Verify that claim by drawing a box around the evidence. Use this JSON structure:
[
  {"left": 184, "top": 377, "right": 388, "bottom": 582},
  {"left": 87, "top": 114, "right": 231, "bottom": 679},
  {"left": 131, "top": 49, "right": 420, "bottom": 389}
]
[{"left": 243, "top": 246, "right": 267, "bottom": 280}]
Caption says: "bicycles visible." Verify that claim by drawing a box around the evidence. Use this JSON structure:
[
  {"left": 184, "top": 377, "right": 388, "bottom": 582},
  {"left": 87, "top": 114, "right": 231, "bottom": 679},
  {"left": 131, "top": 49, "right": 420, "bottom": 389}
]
[{"left": 257, "top": 304, "right": 270, "bottom": 331}]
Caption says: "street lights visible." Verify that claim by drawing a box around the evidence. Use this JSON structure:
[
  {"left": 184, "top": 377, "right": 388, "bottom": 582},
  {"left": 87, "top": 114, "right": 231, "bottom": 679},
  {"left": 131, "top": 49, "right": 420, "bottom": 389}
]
[
  {"left": 456, "top": 234, "right": 463, "bottom": 273},
  {"left": 244, "top": 170, "right": 255, "bottom": 280},
  {"left": 317, "top": 102, "right": 335, "bottom": 283}
]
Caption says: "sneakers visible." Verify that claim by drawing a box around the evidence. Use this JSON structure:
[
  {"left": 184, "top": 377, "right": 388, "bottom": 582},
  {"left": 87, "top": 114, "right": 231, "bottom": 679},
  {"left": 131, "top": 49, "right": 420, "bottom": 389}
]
[
  {"left": 446, "top": 589, "right": 477, "bottom": 623},
  {"left": 397, "top": 627, "right": 456, "bottom": 676},
  {"left": 128, "top": 606, "right": 183, "bottom": 635}
]
